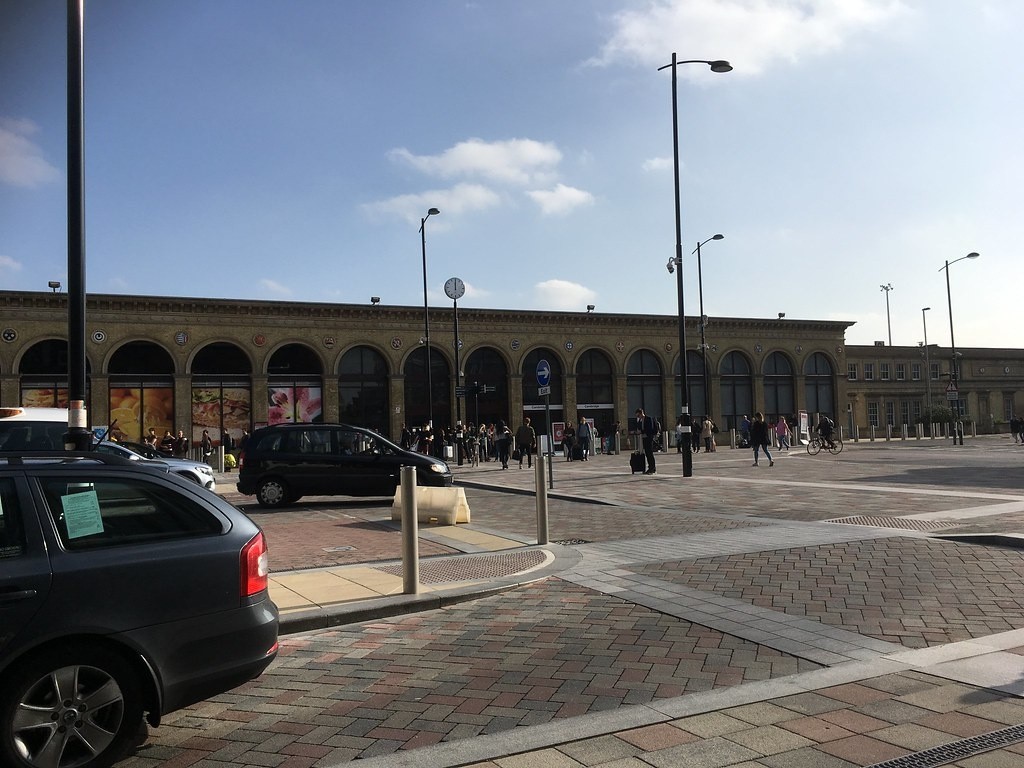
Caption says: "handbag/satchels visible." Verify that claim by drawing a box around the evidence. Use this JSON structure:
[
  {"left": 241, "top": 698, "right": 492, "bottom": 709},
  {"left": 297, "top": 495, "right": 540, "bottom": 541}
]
[
  {"left": 512, "top": 448, "right": 521, "bottom": 460},
  {"left": 205, "top": 451, "right": 211, "bottom": 457},
  {"left": 443, "top": 446, "right": 453, "bottom": 458},
  {"left": 232, "top": 438, "right": 237, "bottom": 450},
  {"left": 714, "top": 426, "right": 719, "bottom": 433}
]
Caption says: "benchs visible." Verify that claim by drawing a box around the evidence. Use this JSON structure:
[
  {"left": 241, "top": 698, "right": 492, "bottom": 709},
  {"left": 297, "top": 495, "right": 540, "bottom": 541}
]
[{"left": 283, "top": 437, "right": 307, "bottom": 453}]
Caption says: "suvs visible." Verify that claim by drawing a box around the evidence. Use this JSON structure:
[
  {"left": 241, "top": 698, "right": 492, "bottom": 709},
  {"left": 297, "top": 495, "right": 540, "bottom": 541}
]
[
  {"left": 237, "top": 421, "right": 454, "bottom": 509},
  {"left": 1, "top": 451, "right": 279, "bottom": 768}
]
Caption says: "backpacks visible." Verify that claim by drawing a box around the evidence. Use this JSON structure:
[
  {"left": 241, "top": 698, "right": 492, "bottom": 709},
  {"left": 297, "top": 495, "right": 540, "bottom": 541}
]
[
  {"left": 825, "top": 417, "right": 834, "bottom": 430},
  {"left": 653, "top": 417, "right": 661, "bottom": 435}
]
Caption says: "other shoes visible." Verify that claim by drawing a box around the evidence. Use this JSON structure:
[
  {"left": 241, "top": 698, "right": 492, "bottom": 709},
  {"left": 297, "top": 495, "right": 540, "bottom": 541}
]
[
  {"left": 693, "top": 449, "right": 699, "bottom": 453},
  {"left": 779, "top": 448, "right": 782, "bottom": 450},
  {"left": 503, "top": 465, "right": 508, "bottom": 470},
  {"left": 753, "top": 462, "right": 760, "bottom": 466},
  {"left": 770, "top": 460, "right": 774, "bottom": 467},
  {"left": 646, "top": 467, "right": 656, "bottom": 475},
  {"left": 787, "top": 445, "right": 789, "bottom": 450},
  {"left": 485, "top": 457, "right": 491, "bottom": 462},
  {"left": 519, "top": 464, "right": 524, "bottom": 470},
  {"left": 704, "top": 449, "right": 709, "bottom": 453}
]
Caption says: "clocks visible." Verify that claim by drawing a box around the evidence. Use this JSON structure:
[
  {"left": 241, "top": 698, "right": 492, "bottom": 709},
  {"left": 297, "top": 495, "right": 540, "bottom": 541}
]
[{"left": 444, "top": 278, "right": 465, "bottom": 299}]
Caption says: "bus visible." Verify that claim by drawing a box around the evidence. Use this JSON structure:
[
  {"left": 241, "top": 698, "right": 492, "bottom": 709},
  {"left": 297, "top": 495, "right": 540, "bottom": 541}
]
[{"left": 0, "top": 406, "right": 88, "bottom": 463}]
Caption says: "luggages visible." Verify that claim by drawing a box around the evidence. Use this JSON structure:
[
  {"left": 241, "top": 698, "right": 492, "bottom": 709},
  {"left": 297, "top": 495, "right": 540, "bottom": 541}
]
[
  {"left": 572, "top": 443, "right": 581, "bottom": 460},
  {"left": 630, "top": 434, "right": 645, "bottom": 473}
]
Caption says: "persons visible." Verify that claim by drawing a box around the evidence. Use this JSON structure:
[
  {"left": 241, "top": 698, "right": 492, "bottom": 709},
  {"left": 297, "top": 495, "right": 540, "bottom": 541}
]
[
  {"left": 814, "top": 415, "right": 836, "bottom": 449},
  {"left": 635, "top": 408, "right": 657, "bottom": 475},
  {"left": 654, "top": 414, "right": 757, "bottom": 453},
  {"left": 776, "top": 415, "right": 793, "bottom": 451},
  {"left": 751, "top": 412, "right": 774, "bottom": 466},
  {"left": 92, "top": 428, "right": 249, "bottom": 472},
  {"left": 606, "top": 421, "right": 621, "bottom": 455},
  {"left": 563, "top": 420, "right": 576, "bottom": 462},
  {"left": 593, "top": 427, "right": 599, "bottom": 454},
  {"left": 342, "top": 417, "right": 537, "bottom": 470},
  {"left": 1010, "top": 414, "right": 1019, "bottom": 443},
  {"left": 1018, "top": 417, "right": 1024, "bottom": 443},
  {"left": 576, "top": 417, "right": 592, "bottom": 461}
]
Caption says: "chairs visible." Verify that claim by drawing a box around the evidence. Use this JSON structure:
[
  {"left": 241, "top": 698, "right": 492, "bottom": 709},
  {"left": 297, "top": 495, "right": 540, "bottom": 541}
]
[{"left": 339, "top": 436, "right": 357, "bottom": 456}]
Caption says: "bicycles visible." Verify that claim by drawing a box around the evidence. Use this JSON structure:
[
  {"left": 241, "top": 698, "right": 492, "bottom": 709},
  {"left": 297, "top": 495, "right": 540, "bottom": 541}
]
[{"left": 807, "top": 433, "right": 844, "bottom": 455}]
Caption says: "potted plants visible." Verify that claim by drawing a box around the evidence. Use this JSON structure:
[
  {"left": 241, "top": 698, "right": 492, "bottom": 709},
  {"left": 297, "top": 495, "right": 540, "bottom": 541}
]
[{"left": 224, "top": 454, "right": 236, "bottom": 472}]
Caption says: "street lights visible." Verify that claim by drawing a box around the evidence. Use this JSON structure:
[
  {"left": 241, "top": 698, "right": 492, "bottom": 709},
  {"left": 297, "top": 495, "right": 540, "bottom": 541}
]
[
  {"left": 672, "top": 60, "right": 733, "bottom": 475},
  {"left": 945, "top": 252, "right": 982, "bottom": 445},
  {"left": 923, "top": 307, "right": 932, "bottom": 439},
  {"left": 423, "top": 207, "right": 440, "bottom": 419},
  {"left": 698, "top": 233, "right": 725, "bottom": 416},
  {"left": 880, "top": 282, "right": 895, "bottom": 346}
]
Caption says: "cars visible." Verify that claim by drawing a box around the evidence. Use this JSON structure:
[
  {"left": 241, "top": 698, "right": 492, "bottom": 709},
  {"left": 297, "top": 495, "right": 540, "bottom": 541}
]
[{"left": 94, "top": 440, "right": 216, "bottom": 500}]
